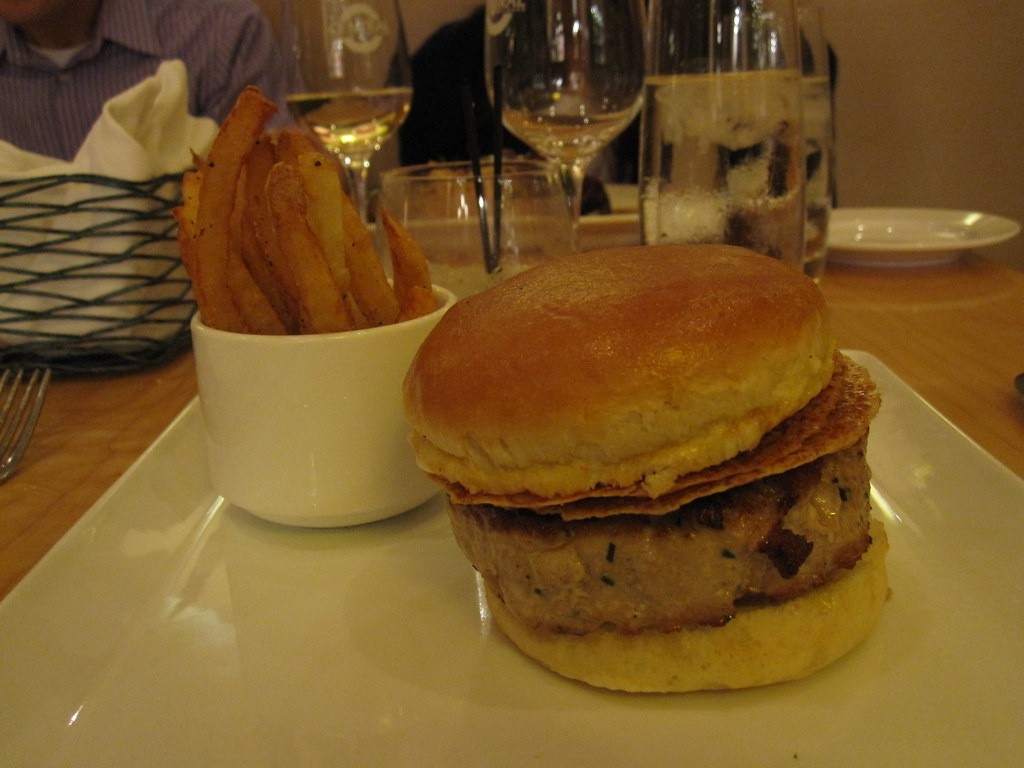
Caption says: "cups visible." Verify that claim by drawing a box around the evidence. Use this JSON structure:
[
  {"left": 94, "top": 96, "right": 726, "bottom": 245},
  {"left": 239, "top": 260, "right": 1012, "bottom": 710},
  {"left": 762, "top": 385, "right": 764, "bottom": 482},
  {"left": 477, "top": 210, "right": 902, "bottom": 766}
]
[
  {"left": 191, "top": 283, "right": 458, "bottom": 529},
  {"left": 639, "top": 0, "right": 806, "bottom": 278},
  {"left": 710, "top": 0, "right": 833, "bottom": 284},
  {"left": 375, "top": 159, "right": 575, "bottom": 305}
]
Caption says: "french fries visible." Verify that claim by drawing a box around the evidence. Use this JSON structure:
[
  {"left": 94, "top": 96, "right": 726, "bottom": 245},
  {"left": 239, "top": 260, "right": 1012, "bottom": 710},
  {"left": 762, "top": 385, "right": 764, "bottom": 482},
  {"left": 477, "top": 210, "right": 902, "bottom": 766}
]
[{"left": 172, "top": 85, "right": 435, "bottom": 336}]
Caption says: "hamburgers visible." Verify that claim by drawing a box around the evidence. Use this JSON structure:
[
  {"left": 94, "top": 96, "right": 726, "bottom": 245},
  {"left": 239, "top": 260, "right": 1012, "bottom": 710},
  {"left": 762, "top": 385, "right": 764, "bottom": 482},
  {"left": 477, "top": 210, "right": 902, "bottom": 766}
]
[{"left": 405, "top": 243, "right": 889, "bottom": 692}]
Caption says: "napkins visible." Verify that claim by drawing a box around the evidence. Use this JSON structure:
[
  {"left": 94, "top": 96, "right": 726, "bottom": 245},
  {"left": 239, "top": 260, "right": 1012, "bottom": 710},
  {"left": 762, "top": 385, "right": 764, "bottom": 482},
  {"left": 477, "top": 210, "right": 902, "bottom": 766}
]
[{"left": 0, "top": 59, "right": 222, "bottom": 347}]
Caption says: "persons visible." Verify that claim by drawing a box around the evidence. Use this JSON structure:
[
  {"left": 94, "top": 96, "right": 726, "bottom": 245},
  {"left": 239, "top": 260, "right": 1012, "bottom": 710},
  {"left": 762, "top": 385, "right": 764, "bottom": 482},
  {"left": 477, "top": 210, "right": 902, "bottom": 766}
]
[
  {"left": 0, "top": 0, "right": 290, "bottom": 161},
  {"left": 396, "top": 0, "right": 838, "bottom": 217}
]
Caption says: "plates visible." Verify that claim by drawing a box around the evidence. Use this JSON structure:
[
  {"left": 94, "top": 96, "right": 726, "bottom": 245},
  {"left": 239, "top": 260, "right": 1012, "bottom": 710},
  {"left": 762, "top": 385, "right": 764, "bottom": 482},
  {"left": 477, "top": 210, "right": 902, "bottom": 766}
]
[
  {"left": 824, "top": 205, "right": 1020, "bottom": 266},
  {"left": 0, "top": 349, "right": 1024, "bottom": 768},
  {"left": 378, "top": 181, "right": 650, "bottom": 251}
]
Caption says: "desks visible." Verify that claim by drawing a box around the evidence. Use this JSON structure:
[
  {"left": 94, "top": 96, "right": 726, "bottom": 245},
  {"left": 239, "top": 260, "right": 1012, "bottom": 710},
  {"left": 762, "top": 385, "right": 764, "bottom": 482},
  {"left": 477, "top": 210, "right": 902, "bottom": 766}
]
[{"left": 0, "top": 254, "right": 1024, "bottom": 598}]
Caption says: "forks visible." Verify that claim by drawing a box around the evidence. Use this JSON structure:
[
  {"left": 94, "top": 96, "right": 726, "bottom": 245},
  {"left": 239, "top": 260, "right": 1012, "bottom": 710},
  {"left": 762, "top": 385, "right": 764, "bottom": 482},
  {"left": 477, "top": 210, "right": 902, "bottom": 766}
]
[{"left": 0, "top": 365, "right": 51, "bottom": 485}]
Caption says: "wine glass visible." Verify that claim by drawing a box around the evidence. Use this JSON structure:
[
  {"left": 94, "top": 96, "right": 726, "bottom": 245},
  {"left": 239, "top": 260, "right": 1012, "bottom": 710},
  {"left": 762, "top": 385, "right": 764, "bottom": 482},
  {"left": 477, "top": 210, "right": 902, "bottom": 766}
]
[
  {"left": 280, "top": 0, "right": 414, "bottom": 223},
  {"left": 484, "top": 0, "right": 647, "bottom": 252}
]
